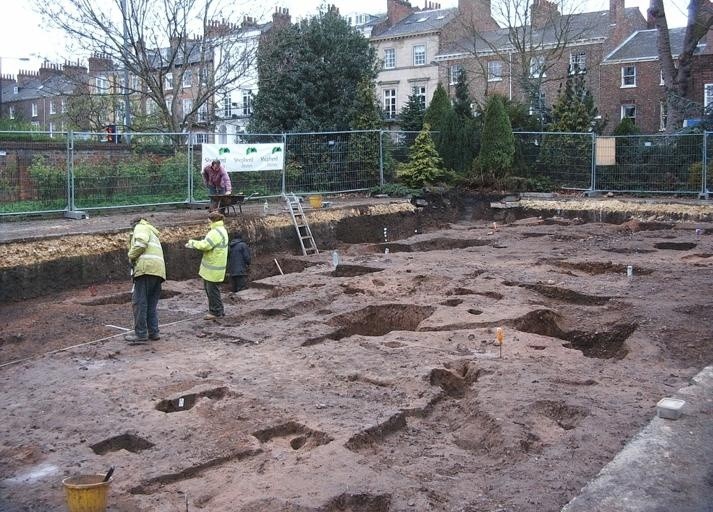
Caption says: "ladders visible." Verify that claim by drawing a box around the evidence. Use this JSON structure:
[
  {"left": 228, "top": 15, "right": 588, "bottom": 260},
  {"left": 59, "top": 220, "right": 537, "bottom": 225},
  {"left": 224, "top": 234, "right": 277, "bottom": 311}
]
[{"left": 281, "top": 192, "right": 320, "bottom": 256}]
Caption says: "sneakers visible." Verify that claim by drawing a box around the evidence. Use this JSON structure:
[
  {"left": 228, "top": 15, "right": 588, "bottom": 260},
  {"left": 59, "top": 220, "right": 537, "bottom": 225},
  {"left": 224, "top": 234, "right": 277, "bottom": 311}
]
[
  {"left": 150, "top": 334, "right": 160, "bottom": 340},
  {"left": 204, "top": 312, "right": 224, "bottom": 321},
  {"left": 125, "top": 334, "right": 148, "bottom": 341}
]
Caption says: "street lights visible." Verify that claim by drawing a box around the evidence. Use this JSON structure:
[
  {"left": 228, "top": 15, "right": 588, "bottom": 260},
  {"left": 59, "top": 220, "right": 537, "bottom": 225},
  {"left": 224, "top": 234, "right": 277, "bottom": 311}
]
[
  {"left": 0, "top": 56, "right": 30, "bottom": 105},
  {"left": 430, "top": 60, "right": 450, "bottom": 103}
]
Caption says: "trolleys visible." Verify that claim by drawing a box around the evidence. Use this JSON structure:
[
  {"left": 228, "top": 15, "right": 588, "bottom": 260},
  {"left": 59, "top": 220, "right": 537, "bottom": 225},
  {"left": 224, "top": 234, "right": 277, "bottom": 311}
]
[{"left": 208, "top": 191, "right": 260, "bottom": 215}]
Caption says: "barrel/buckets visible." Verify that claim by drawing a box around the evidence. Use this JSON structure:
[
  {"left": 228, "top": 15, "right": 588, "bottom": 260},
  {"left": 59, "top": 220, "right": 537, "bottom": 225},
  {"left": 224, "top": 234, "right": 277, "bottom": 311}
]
[
  {"left": 61, "top": 472, "right": 115, "bottom": 512},
  {"left": 309, "top": 195, "right": 322, "bottom": 208}
]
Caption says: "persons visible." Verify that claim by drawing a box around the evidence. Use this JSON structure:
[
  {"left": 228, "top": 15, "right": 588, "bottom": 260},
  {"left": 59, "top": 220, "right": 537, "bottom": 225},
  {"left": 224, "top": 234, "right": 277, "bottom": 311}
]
[
  {"left": 124, "top": 212, "right": 168, "bottom": 342},
  {"left": 229, "top": 232, "right": 253, "bottom": 293},
  {"left": 200, "top": 160, "right": 234, "bottom": 216},
  {"left": 185, "top": 210, "right": 231, "bottom": 321}
]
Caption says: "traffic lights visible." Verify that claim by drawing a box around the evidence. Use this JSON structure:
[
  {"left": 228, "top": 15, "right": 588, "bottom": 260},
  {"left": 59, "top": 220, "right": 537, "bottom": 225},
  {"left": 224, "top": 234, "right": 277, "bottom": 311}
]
[{"left": 106, "top": 125, "right": 117, "bottom": 143}]
